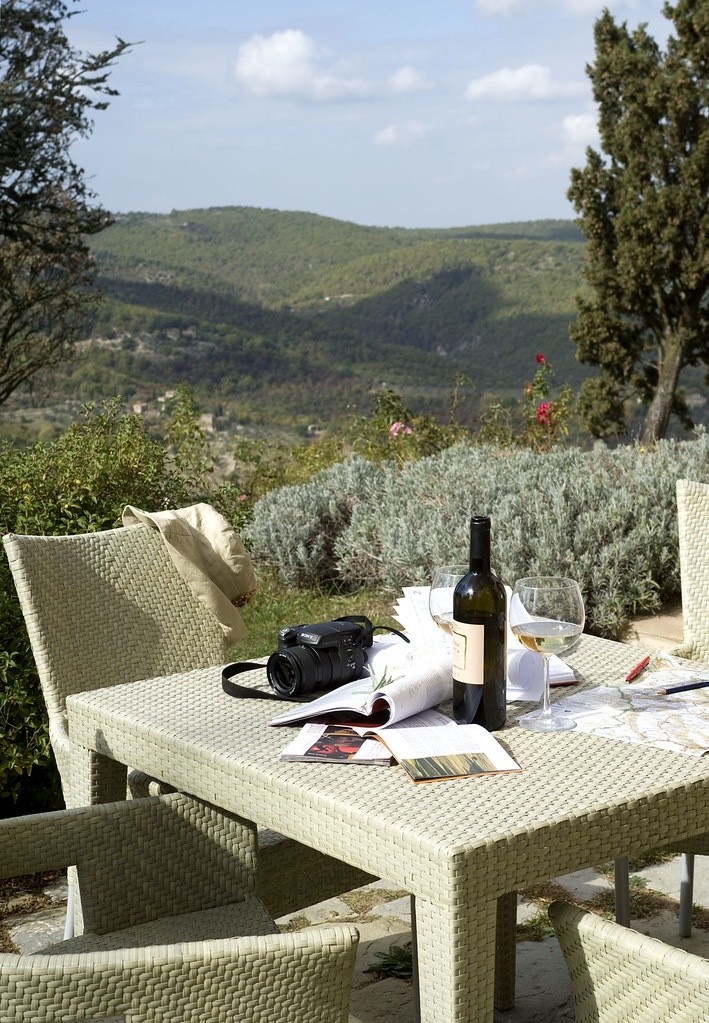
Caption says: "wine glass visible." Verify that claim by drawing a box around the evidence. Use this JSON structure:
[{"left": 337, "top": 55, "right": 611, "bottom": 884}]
[
  {"left": 509, "top": 576, "right": 585, "bottom": 732},
  {"left": 429, "top": 565, "right": 496, "bottom": 713}
]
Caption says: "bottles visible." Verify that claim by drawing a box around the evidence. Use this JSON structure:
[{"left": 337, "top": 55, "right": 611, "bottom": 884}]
[{"left": 452, "top": 517, "right": 507, "bottom": 731}]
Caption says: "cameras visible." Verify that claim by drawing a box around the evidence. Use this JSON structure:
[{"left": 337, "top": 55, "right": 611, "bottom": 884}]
[{"left": 266, "top": 621, "right": 366, "bottom": 697}]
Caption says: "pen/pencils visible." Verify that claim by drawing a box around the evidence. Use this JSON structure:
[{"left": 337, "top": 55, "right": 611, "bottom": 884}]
[{"left": 657, "top": 680, "right": 709, "bottom": 695}]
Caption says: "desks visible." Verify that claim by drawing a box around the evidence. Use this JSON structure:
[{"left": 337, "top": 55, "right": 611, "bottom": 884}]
[{"left": 65, "top": 633, "right": 709, "bottom": 1023}]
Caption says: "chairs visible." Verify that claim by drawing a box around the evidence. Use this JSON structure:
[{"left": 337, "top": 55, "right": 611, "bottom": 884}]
[
  {"left": 1, "top": 521, "right": 381, "bottom": 941},
  {"left": 651, "top": 479, "right": 709, "bottom": 938},
  {"left": 547, "top": 900, "right": 709, "bottom": 1023},
  {"left": 0, "top": 789, "right": 361, "bottom": 1023}
]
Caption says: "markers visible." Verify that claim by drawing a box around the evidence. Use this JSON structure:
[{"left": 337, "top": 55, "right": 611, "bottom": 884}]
[{"left": 624, "top": 655, "right": 651, "bottom": 682}]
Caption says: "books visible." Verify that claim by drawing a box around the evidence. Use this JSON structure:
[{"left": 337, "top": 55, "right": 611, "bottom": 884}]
[
  {"left": 270, "top": 671, "right": 521, "bottom": 784},
  {"left": 389, "top": 587, "right": 577, "bottom": 686}
]
